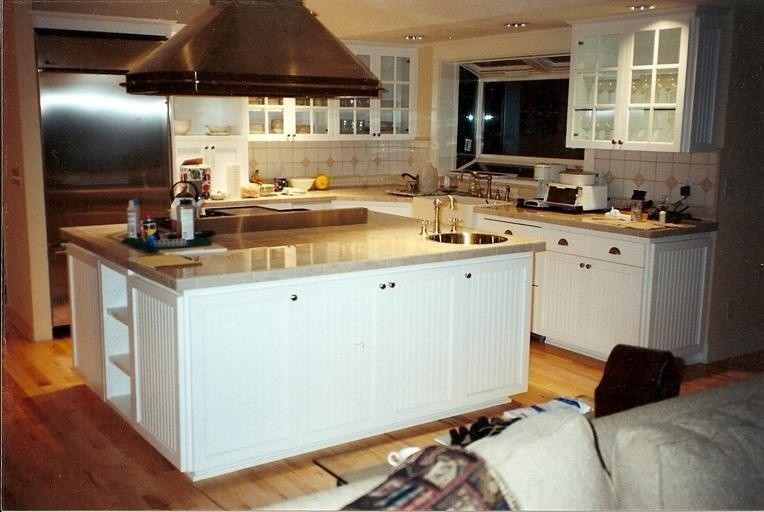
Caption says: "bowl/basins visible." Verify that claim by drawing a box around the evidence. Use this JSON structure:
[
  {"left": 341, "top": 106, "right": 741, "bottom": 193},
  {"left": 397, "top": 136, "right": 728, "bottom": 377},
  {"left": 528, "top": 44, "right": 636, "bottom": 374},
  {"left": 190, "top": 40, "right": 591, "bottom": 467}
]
[
  {"left": 340, "top": 119, "right": 366, "bottom": 132},
  {"left": 249, "top": 123, "right": 265, "bottom": 134},
  {"left": 172, "top": 118, "right": 192, "bottom": 136},
  {"left": 381, "top": 119, "right": 392, "bottom": 132},
  {"left": 289, "top": 177, "right": 315, "bottom": 192}
]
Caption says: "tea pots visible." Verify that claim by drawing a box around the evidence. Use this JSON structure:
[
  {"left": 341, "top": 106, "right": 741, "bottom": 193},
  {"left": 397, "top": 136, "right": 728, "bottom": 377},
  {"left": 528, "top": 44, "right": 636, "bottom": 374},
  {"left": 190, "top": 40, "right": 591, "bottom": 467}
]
[
  {"left": 168, "top": 178, "right": 201, "bottom": 222},
  {"left": 202, "top": 121, "right": 230, "bottom": 133}
]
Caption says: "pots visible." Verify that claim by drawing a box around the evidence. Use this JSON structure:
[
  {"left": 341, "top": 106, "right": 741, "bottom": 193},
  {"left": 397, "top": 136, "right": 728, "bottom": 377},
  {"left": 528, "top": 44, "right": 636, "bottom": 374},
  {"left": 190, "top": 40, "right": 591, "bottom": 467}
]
[{"left": 558, "top": 164, "right": 597, "bottom": 185}]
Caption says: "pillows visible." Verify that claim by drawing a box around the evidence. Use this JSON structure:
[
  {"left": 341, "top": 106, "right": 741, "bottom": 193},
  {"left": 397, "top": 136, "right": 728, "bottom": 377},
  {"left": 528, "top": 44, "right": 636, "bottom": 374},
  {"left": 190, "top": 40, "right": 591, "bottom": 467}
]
[
  {"left": 337, "top": 443, "right": 523, "bottom": 511},
  {"left": 464, "top": 405, "right": 615, "bottom": 510},
  {"left": 612, "top": 400, "right": 763, "bottom": 511}
]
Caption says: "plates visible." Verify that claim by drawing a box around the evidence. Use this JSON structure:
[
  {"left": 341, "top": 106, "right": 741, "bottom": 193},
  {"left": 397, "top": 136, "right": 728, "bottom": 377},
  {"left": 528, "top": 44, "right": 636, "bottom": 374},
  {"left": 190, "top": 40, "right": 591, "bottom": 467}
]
[
  {"left": 205, "top": 131, "right": 230, "bottom": 137},
  {"left": 225, "top": 163, "right": 242, "bottom": 200}
]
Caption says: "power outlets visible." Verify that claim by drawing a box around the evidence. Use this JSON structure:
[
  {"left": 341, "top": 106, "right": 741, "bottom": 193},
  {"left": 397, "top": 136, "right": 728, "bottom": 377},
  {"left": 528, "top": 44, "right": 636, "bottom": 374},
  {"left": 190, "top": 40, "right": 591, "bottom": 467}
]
[{"left": 680, "top": 186, "right": 690, "bottom": 196}]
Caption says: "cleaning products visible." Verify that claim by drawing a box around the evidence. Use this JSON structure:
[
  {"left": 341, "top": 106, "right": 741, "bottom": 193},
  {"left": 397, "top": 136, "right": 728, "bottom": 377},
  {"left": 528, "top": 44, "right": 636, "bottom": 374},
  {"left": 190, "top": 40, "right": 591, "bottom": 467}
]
[{"left": 175, "top": 199, "right": 197, "bottom": 244}]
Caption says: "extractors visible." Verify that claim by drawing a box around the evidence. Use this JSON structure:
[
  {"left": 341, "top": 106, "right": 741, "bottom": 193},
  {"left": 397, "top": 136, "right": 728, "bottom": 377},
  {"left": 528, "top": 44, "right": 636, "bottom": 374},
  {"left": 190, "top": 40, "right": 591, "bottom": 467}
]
[{"left": 122, "top": 0, "right": 388, "bottom": 100}]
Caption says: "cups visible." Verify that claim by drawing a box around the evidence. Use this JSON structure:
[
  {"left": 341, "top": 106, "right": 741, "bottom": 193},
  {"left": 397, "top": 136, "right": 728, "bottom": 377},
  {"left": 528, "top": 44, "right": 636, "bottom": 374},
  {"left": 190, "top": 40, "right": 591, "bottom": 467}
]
[
  {"left": 388, "top": 447, "right": 420, "bottom": 468},
  {"left": 261, "top": 176, "right": 288, "bottom": 196},
  {"left": 630, "top": 199, "right": 643, "bottom": 222}
]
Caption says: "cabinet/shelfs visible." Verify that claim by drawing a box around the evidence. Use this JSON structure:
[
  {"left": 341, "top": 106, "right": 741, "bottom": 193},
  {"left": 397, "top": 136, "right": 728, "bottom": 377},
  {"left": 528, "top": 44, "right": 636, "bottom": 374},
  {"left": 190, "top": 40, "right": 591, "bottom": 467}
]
[
  {"left": 475, "top": 213, "right": 544, "bottom": 344},
  {"left": 248, "top": 34, "right": 419, "bottom": 142},
  {"left": 532, "top": 223, "right": 714, "bottom": 363},
  {"left": 60, "top": 240, "right": 534, "bottom": 484},
  {"left": 565, "top": 9, "right": 729, "bottom": 155},
  {"left": 169, "top": 95, "right": 249, "bottom": 198}
]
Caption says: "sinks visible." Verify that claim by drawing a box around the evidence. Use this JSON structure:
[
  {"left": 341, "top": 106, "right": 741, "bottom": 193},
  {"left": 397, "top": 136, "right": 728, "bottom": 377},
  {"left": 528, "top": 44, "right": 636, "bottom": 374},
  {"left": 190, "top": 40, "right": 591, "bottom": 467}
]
[
  {"left": 387, "top": 190, "right": 420, "bottom": 196},
  {"left": 413, "top": 195, "right": 508, "bottom": 206},
  {"left": 426, "top": 234, "right": 511, "bottom": 245}
]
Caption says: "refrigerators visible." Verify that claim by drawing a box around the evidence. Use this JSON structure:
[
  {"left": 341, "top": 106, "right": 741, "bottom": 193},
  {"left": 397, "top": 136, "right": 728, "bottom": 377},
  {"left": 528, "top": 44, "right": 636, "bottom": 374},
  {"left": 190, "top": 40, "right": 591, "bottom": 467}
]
[{"left": 33, "top": 27, "right": 175, "bottom": 328}]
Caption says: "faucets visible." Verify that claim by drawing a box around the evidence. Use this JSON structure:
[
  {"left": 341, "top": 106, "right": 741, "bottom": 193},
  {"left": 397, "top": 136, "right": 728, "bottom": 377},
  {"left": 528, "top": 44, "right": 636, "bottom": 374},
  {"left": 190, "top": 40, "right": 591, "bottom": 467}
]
[
  {"left": 401, "top": 173, "right": 419, "bottom": 190},
  {"left": 434, "top": 194, "right": 457, "bottom": 233},
  {"left": 460, "top": 169, "right": 492, "bottom": 197}
]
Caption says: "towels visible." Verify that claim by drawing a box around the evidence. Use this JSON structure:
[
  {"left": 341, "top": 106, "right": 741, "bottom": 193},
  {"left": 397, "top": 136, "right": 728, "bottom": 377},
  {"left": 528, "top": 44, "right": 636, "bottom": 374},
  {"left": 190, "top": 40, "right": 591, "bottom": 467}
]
[{"left": 598, "top": 219, "right": 666, "bottom": 231}]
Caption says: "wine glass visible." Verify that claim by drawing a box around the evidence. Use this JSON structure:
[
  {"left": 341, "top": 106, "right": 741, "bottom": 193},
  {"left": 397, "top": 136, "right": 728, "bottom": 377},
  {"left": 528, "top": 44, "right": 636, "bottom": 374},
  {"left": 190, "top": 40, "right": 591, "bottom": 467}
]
[{"left": 583, "top": 73, "right": 677, "bottom": 145}]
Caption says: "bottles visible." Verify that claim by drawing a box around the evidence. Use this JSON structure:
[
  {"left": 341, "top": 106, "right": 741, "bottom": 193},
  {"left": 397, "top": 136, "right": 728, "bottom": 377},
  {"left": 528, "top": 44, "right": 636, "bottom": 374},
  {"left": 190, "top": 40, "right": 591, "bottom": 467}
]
[
  {"left": 659, "top": 207, "right": 666, "bottom": 224},
  {"left": 175, "top": 200, "right": 199, "bottom": 241},
  {"left": 272, "top": 119, "right": 282, "bottom": 133},
  {"left": 124, "top": 196, "right": 158, "bottom": 245},
  {"left": 418, "top": 159, "right": 440, "bottom": 195}
]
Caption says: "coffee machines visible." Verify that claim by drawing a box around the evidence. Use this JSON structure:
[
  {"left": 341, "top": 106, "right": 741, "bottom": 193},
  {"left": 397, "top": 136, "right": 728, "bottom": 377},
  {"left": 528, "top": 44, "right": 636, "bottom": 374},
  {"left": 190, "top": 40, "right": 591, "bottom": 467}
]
[{"left": 524, "top": 163, "right": 568, "bottom": 208}]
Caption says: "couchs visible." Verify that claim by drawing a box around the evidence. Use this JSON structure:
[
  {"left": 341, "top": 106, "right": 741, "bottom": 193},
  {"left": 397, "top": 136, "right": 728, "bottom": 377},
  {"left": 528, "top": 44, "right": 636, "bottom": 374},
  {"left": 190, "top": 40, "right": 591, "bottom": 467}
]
[{"left": 258, "top": 377, "right": 764, "bottom": 510}]
